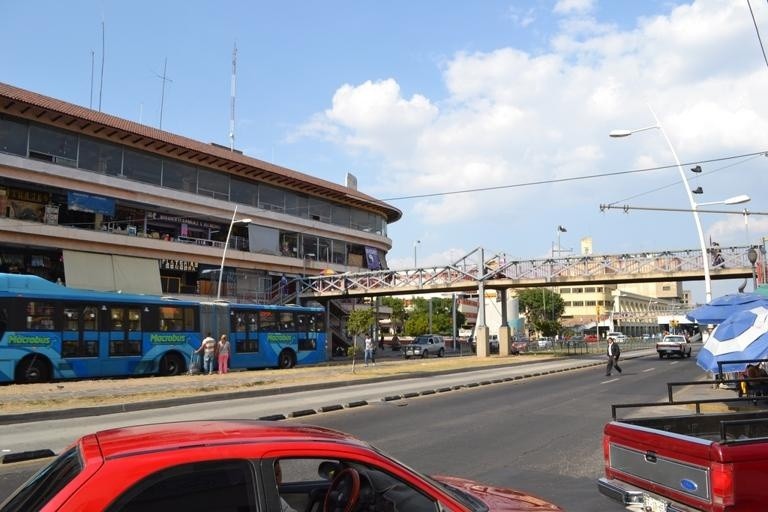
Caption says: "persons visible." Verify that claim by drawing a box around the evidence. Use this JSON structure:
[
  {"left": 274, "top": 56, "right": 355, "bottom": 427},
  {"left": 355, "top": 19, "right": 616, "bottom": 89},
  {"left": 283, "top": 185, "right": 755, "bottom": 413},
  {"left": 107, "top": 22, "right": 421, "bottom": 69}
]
[
  {"left": 216, "top": 334, "right": 232, "bottom": 374},
  {"left": 710, "top": 242, "right": 728, "bottom": 269},
  {"left": 194, "top": 333, "right": 216, "bottom": 374},
  {"left": 604, "top": 338, "right": 622, "bottom": 376},
  {"left": 737, "top": 365, "right": 768, "bottom": 401},
  {"left": 274, "top": 461, "right": 298, "bottom": 512},
  {"left": 363, "top": 333, "right": 377, "bottom": 367}
]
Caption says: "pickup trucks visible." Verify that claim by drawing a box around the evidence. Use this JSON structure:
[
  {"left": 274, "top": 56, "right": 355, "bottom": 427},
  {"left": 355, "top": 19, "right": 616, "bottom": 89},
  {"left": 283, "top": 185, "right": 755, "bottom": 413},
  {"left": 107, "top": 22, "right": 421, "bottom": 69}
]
[{"left": 656, "top": 333, "right": 692, "bottom": 360}]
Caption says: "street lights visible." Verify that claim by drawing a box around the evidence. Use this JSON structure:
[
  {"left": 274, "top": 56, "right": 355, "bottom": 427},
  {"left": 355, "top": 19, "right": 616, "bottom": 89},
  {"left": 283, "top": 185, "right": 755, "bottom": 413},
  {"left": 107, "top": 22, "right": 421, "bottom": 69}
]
[
  {"left": 214, "top": 204, "right": 252, "bottom": 300},
  {"left": 609, "top": 123, "right": 752, "bottom": 389}
]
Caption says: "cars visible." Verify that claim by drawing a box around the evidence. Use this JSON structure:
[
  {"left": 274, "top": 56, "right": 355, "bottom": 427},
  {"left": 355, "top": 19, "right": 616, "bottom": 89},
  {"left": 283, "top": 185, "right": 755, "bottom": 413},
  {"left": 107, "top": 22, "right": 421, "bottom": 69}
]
[
  {"left": 0, "top": 415, "right": 563, "bottom": 512},
  {"left": 470, "top": 332, "right": 631, "bottom": 353}
]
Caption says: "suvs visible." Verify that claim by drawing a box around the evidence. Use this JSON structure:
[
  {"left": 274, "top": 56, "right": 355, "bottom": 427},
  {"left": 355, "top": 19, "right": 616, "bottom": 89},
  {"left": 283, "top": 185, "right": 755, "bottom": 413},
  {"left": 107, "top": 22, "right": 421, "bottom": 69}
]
[{"left": 400, "top": 332, "right": 446, "bottom": 358}]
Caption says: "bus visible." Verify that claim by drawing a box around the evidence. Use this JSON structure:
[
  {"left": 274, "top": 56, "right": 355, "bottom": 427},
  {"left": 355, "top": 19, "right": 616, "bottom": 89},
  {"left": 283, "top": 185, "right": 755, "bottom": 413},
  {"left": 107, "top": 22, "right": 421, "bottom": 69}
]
[{"left": 0, "top": 271, "right": 331, "bottom": 385}]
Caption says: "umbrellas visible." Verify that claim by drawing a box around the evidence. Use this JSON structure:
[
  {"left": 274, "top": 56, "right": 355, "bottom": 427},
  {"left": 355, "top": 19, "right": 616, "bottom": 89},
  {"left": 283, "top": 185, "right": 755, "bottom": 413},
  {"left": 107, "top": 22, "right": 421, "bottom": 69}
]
[{"left": 686, "top": 291, "right": 768, "bottom": 375}]
